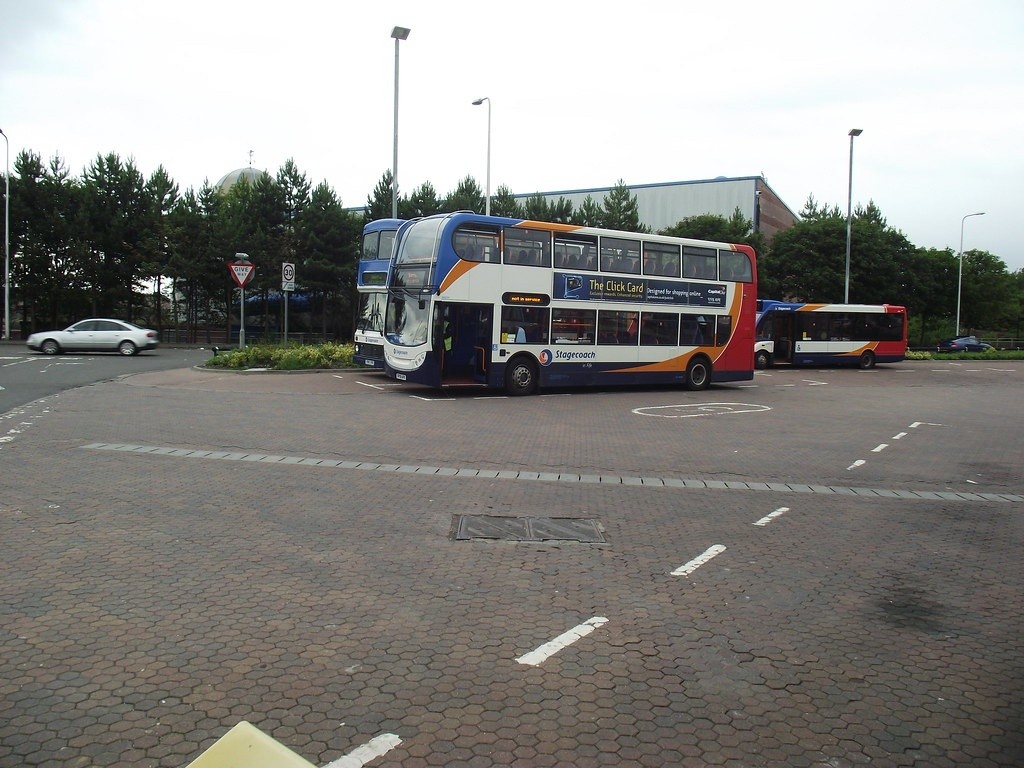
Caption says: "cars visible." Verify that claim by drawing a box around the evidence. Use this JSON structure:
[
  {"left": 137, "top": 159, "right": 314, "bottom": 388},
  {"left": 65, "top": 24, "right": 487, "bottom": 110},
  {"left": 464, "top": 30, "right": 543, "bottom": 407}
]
[
  {"left": 936, "top": 336, "right": 995, "bottom": 353},
  {"left": 25, "top": 317, "right": 161, "bottom": 356}
]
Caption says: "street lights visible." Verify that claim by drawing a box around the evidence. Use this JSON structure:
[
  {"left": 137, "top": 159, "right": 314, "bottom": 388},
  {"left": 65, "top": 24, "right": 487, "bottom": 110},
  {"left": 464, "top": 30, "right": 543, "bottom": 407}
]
[
  {"left": 472, "top": 97, "right": 491, "bottom": 262},
  {"left": 389, "top": 24, "right": 411, "bottom": 219},
  {"left": 954, "top": 212, "right": 985, "bottom": 337},
  {"left": 844, "top": 128, "right": 863, "bottom": 305}
]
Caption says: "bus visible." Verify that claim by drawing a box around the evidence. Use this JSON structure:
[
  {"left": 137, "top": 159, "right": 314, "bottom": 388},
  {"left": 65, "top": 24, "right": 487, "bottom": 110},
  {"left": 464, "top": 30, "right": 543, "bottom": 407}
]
[
  {"left": 351, "top": 208, "right": 758, "bottom": 397},
  {"left": 753, "top": 298, "right": 908, "bottom": 370}
]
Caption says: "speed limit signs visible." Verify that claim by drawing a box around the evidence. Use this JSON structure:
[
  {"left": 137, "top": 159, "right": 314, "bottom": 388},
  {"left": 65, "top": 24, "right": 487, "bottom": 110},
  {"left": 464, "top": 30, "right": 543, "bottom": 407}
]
[{"left": 281, "top": 262, "right": 296, "bottom": 291}]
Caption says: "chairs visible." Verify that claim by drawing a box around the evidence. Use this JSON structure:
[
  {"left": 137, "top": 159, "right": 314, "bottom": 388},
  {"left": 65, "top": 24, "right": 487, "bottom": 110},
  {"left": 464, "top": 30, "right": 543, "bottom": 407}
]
[
  {"left": 450, "top": 245, "right": 733, "bottom": 279},
  {"left": 616, "top": 320, "right": 704, "bottom": 344}
]
